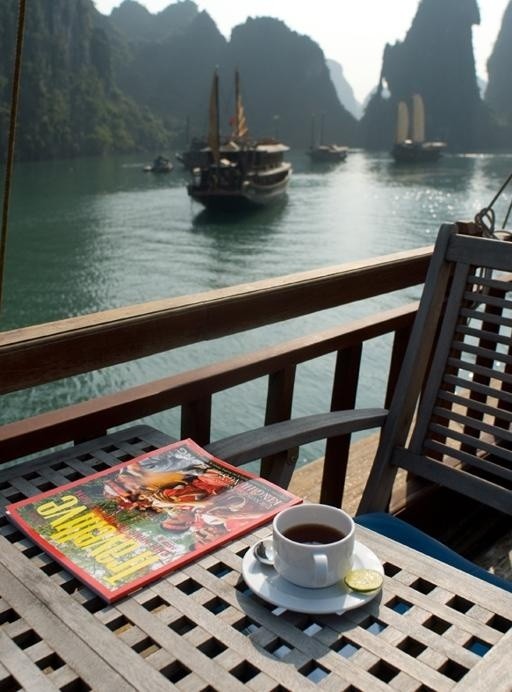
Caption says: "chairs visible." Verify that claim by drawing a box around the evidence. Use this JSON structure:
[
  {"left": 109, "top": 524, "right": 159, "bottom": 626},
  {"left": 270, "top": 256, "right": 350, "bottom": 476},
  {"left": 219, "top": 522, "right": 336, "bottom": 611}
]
[{"left": 201, "top": 221, "right": 512, "bottom": 692}]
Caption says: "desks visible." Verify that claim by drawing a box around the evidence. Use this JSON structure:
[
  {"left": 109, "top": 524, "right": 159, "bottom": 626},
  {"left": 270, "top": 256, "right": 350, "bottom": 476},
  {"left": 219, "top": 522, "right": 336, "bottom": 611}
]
[{"left": 0, "top": 415, "right": 512, "bottom": 692}]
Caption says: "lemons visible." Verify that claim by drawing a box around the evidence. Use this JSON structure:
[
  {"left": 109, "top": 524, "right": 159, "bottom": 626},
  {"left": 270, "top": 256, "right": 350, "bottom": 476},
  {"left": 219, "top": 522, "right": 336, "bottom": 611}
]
[{"left": 345, "top": 568, "right": 384, "bottom": 592}]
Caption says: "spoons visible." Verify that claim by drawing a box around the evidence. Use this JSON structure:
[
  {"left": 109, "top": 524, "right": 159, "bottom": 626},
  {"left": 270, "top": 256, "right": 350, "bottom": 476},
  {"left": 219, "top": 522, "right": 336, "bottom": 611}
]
[{"left": 253, "top": 541, "right": 274, "bottom": 567}]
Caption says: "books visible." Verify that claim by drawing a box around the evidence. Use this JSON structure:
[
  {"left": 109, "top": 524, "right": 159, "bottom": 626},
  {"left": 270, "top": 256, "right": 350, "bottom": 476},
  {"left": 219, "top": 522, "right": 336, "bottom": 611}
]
[{"left": 3, "top": 437, "right": 303, "bottom": 605}]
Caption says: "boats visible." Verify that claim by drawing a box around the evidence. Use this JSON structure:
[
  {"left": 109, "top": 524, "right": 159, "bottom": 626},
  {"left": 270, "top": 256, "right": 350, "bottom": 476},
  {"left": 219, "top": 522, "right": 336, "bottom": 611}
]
[
  {"left": 179, "top": 118, "right": 243, "bottom": 168},
  {"left": 306, "top": 111, "right": 350, "bottom": 162},
  {"left": 145, "top": 155, "right": 176, "bottom": 175}
]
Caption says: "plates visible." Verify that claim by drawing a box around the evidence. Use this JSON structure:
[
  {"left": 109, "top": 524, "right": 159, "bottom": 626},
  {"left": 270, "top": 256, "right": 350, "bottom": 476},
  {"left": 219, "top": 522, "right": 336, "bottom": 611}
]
[{"left": 240, "top": 536, "right": 386, "bottom": 614}]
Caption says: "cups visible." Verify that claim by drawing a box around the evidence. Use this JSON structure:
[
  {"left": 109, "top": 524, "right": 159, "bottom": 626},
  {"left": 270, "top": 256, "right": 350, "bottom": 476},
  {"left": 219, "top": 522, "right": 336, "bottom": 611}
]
[{"left": 274, "top": 503, "right": 355, "bottom": 592}]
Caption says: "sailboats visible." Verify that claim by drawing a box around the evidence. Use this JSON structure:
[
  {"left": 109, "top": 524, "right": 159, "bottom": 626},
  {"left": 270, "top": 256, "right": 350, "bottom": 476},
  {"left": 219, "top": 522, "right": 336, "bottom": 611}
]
[
  {"left": 386, "top": 90, "right": 449, "bottom": 163},
  {"left": 184, "top": 62, "right": 295, "bottom": 212}
]
